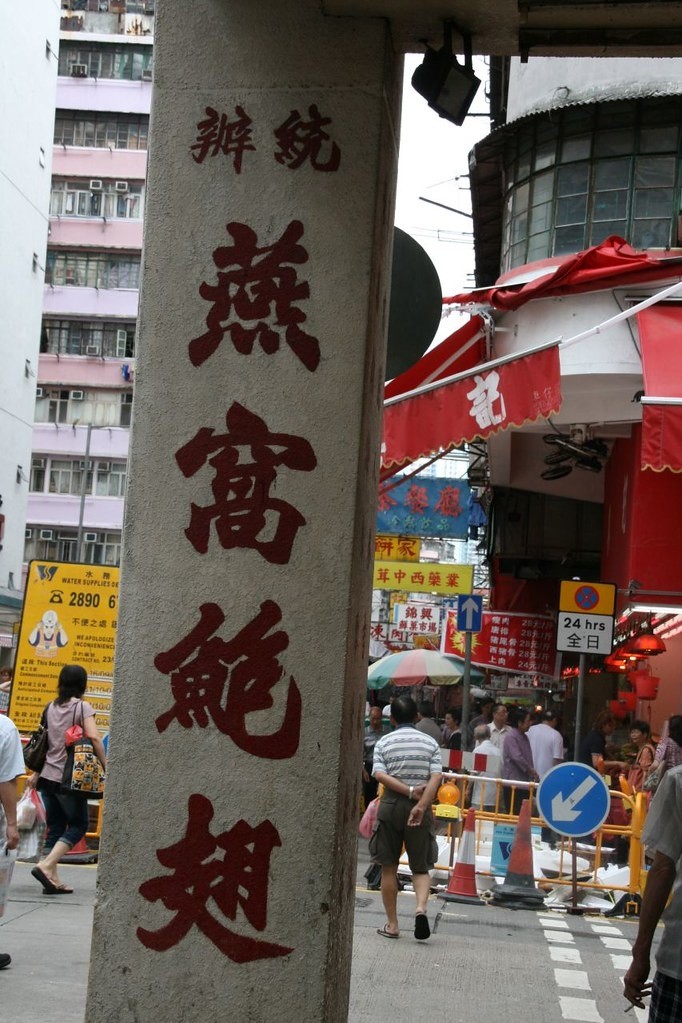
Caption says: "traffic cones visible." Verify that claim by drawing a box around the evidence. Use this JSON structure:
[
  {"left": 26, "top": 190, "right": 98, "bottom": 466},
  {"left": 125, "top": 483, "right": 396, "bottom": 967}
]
[
  {"left": 489, "top": 797, "right": 550, "bottom": 911},
  {"left": 435, "top": 807, "right": 489, "bottom": 907}
]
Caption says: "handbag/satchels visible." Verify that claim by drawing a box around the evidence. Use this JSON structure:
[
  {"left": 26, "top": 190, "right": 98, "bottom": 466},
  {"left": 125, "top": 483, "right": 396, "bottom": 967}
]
[
  {"left": 13, "top": 785, "right": 47, "bottom": 863},
  {"left": 644, "top": 738, "right": 670, "bottom": 790},
  {"left": 627, "top": 746, "right": 654, "bottom": 795},
  {"left": 61, "top": 699, "right": 105, "bottom": 799},
  {"left": 358, "top": 795, "right": 379, "bottom": 839},
  {"left": 23, "top": 702, "right": 52, "bottom": 773}
]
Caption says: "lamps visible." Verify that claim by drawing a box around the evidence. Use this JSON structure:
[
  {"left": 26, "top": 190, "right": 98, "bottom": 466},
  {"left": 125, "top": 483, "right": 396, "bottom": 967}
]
[
  {"left": 411, "top": 22, "right": 482, "bottom": 125},
  {"left": 467, "top": 454, "right": 490, "bottom": 488},
  {"left": 604, "top": 613, "right": 667, "bottom": 670}
]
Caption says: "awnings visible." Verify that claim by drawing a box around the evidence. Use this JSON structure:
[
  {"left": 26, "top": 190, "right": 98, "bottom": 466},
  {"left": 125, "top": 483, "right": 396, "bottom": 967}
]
[{"left": 637, "top": 300, "right": 682, "bottom": 472}]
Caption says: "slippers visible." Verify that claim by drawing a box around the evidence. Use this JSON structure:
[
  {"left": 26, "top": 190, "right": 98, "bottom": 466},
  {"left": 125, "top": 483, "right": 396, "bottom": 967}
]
[
  {"left": 30, "top": 865, "right": 59, "bottom": 894},
  {"left": 42, "top": 883, "right": 73, "bottom": 894},
  {"left": 376, "top": 923, "right": 399, "bottom": 938},
  {"left": 414, "top": 913, "right": 431, "bottom": 939}
]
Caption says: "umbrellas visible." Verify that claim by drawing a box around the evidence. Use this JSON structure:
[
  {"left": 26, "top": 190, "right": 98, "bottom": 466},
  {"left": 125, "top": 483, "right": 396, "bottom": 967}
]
[{"left": 366, "top": 649, "right": 485, "bottom": 702}]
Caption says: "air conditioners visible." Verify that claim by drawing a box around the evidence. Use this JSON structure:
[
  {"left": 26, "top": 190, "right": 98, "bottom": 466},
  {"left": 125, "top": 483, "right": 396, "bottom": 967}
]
[
  {"left": 79, "top": 461, "right": 92, "bottom": 470},
  {"left": 25, "top": 529, "right": 32, "bottom": 538},
  {"left": 70, "top": 389, "right": 84, "bottom": 400},
  {"left": 89, "top": 180, "right": 104, "bottom": 191},
  {"left": 96, "top": 461, "right": 110, "bottom": 472},
  {"left": 39, "top": 529, "right": 53, "bottom": 541},
  {"left": 31, "top": 458, "right": 44, "bottom": 469},
  {"left": 83, "top": 532, "right": 97, "bottom": 543},
  {"left": 36, "top": 387, "right": 46, "bottom": 399},
  {"left": 140, "top": 69, "right": 152, "bottom": 81},
  {"left": 114, "top": 180, "right": 129, "bottom": 192},
  {"left": 86, "top": 346, "right": 99, "bottom": 356},
  {"left": 70, "top": 63, "right": 89, "bottom": 78}
]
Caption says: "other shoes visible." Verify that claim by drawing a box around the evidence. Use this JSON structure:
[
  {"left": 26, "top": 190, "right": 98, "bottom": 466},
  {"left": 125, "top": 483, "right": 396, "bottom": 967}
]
[{"left": 0, "top": 953, "right": 11, "bottom": 969}]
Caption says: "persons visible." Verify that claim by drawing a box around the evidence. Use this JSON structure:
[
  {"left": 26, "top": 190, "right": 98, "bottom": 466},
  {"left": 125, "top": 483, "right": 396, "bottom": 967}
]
[
  {"left": 649, "top": 715, "right": 682, "bottom": 778},
  {"left": 578, "top": 710, "right": 628, "bottom": 770},
  {"left": 31, "top": 664, "right": 106, "bottom": 893},
  {"left": 363, "top": 707, "right": 392, "bottom": 808},
  {"left": 626, "top": 721, "right": 655, "bottom": 792},
  {"left": 382, "top": 695, "right": 394, "bottom": 717},
  {"left": 488, "top": 703, "right": 510, "bottom": 748},
  {"left": 503, "top": 707, "right": 538, "bottom": 815},
  {"left": 372, "top": 694, "right": 441, "bottom": 939},
  {"left": 468, "top": 697, "right": 494, "bottom": 750},
  {"left": 416, "top": 700, "right": 441, "bottom": 744},
  {"left": 623, "top": 764, "right": 682, "bottom": 1023},
  {"left": 0, "top": 666, "right": 13, "bottom": 716},
  {"left": 440, "top": 705, "right": 473, "bottom": 752},
  {"left": 465, "top": 724, "right": 504, "bottom": 811},
  {"left": 0, "top": 714, "right": 25, "bottom": 969},
  {"left": 524, "top": 709, "right": 564, "bottom": 780}
]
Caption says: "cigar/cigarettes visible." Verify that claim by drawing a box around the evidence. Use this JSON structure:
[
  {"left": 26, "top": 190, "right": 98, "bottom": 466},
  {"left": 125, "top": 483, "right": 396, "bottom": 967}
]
[{"left": 625, "top": 998, "right": 642, "bottom": 1013}]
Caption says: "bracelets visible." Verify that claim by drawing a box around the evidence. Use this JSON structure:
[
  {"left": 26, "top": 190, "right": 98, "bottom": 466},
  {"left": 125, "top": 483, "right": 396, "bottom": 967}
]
[{"left": 409, "top": 786, "right": 413, "bottom": 800}]
